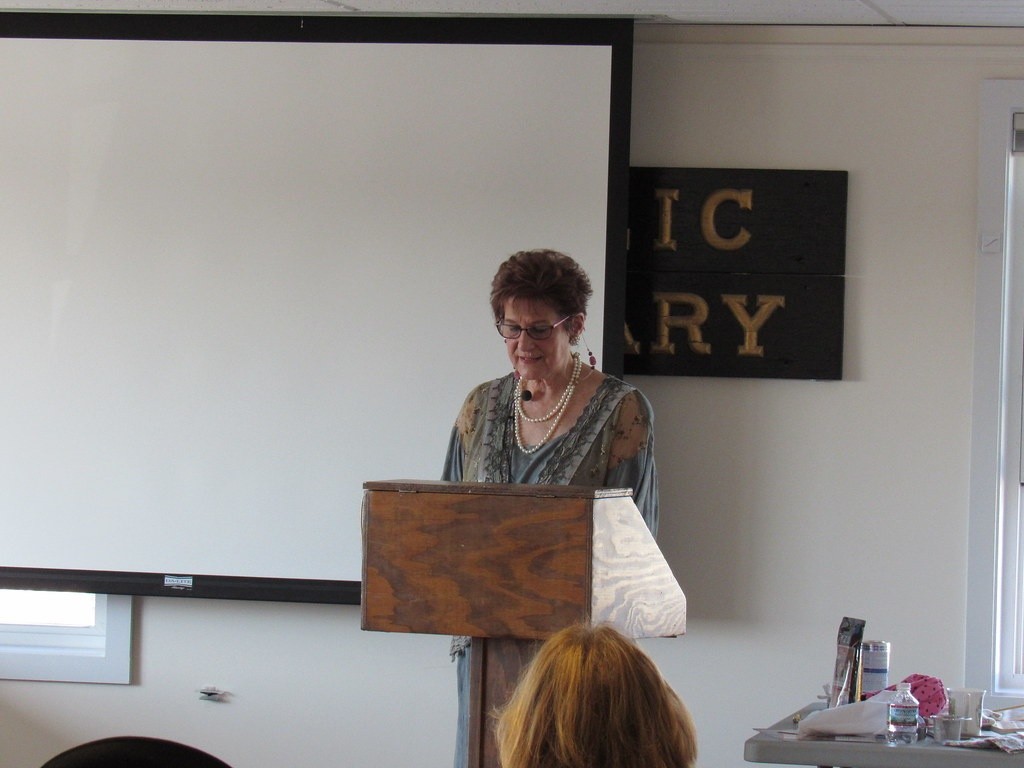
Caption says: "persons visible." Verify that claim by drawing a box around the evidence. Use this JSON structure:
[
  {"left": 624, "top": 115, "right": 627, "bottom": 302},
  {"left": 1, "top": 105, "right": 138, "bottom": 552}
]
[
  {"left": 440, "top": 249, "right": 661, "bottom": 768},
  {"left": 494, "top": 620, "right": 698, "bottom": 768}
]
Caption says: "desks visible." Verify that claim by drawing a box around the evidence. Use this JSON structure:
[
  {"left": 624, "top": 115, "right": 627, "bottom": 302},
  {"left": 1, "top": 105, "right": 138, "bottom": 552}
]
[{"left": 744, "top": 701, "right": 1024, "bottom": 768}]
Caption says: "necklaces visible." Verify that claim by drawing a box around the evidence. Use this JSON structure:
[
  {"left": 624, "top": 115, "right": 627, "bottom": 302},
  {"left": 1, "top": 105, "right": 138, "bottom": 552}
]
[{"left": 514, "top": 350, "right": 582, "bottom": 454}]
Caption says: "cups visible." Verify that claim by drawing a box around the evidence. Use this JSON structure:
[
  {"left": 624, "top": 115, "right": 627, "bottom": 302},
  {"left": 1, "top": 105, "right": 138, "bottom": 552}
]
[
  {"left": 930, "top": 714, "right": 972, "bottom": 745},
  {"left": 947, "top": 688, "right": 985, "bottom": 735}
]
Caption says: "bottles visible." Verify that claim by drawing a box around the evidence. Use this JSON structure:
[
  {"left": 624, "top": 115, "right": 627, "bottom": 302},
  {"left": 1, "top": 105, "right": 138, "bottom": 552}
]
[
  {"left": 886, "top": 682, "right": 919, "bottom": 745},
  {"left": 862, "top": 640, "right": 890, "bottom": 696}
]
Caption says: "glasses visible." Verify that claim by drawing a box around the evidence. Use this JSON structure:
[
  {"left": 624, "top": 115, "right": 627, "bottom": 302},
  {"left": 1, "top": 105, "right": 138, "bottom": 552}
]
[{"left": 496, "top": 313, "right": 573, "bottom": 341}]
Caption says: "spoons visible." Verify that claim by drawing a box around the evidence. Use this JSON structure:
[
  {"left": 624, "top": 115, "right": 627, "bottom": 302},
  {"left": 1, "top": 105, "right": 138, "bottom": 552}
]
[{"left": 928, "top": 730, "right": 996, "bottom": 739}]
